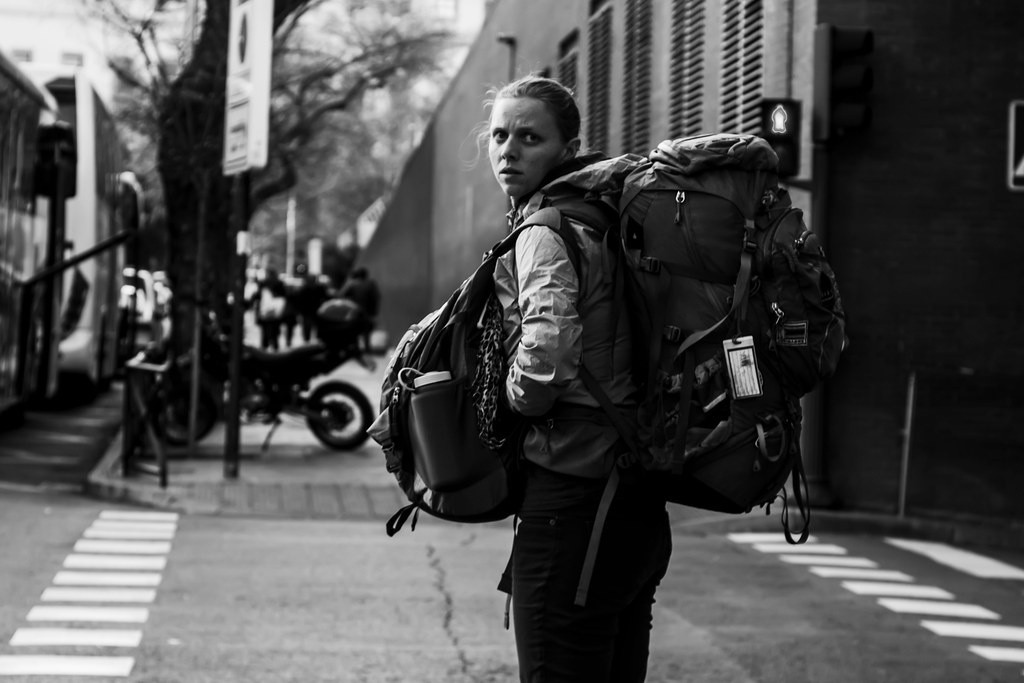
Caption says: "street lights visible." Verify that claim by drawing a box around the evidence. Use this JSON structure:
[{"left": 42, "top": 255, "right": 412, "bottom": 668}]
[{"left": 497, "top": 33, "right": 517, "bottom": 235}]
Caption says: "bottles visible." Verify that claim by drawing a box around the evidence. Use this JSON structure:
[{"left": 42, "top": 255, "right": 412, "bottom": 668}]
[{"left": 399, "top": 365, "right": 453, "bottom": 397}]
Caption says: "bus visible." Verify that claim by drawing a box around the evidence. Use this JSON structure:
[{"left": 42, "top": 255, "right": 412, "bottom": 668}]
[
  {"left": 1, "top": 52, "right": 73, "bottom": 418},
  {"left": 36, "top": 70, "right": 134, "bottom": 405}
]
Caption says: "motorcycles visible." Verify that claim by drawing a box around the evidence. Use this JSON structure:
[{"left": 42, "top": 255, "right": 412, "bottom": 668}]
[{"left": 146, "top": 285, "right": 374, "bottom": 452}]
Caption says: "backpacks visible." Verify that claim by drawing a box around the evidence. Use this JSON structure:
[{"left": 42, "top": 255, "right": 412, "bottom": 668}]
[
  {"left": 365, "top": 206, "right": 582, "bottom": 630},
  {"left": 551, "top": 133, "right": 849, "bottom": 606}
]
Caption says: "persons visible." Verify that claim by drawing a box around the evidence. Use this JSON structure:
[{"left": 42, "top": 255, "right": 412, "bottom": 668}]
[
  {"left": 480, "top": 75, "right": 672, "bottom": 683},
  {"left": 255, "top": 265, "right": 379, "bottom": 354}
]
